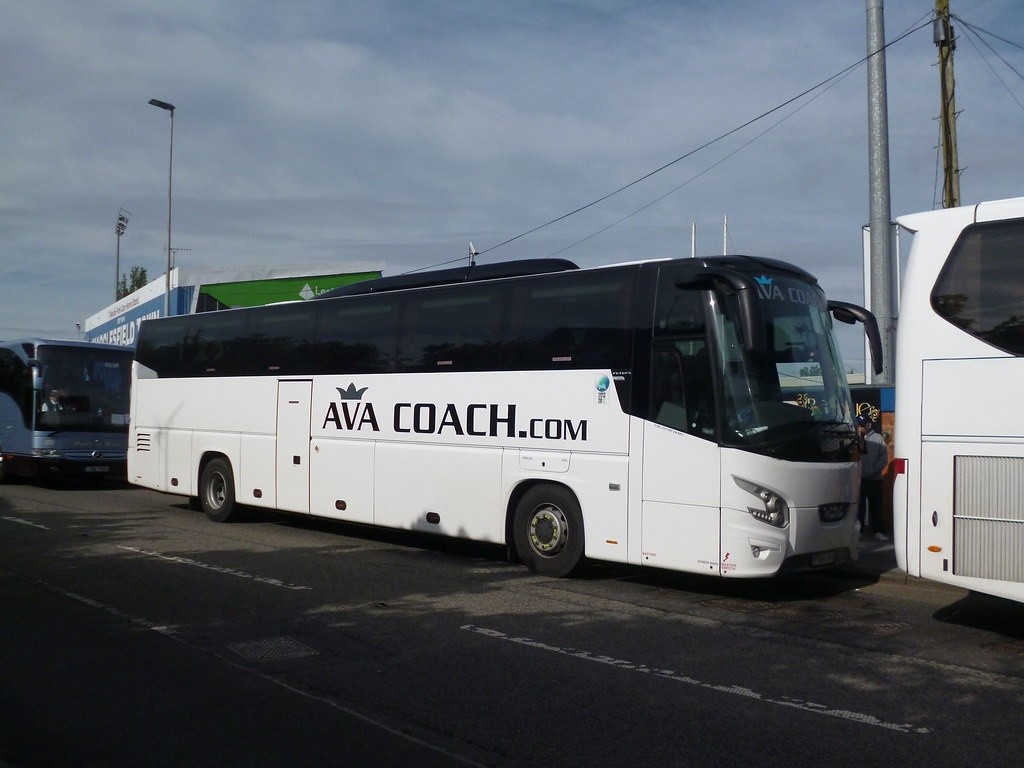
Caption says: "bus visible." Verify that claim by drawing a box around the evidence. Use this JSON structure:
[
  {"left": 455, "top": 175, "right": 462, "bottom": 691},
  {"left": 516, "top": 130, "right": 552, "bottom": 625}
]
[
  {"left": 125, "top": 257, "right": 885, "bottom": 581},
  {"left": 0, "top": 338, "right": 135, "bottom": 480},
  {"left": 893, "top": 197, "right": 1024, "bottom": 606}
]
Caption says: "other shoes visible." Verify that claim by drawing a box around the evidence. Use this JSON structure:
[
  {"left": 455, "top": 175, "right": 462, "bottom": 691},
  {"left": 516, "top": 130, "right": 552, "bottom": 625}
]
[{"left": 874, "top": 531, "right": 889, "bottom": 541}]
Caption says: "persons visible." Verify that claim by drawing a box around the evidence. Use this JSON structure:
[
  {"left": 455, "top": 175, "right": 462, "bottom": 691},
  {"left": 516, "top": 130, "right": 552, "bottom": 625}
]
[
  {"left": 42, "top": 389, "right": 64, "bottom": 412},
  {"left": 857, "top": 414, "right": 889, "bottom": 542}
]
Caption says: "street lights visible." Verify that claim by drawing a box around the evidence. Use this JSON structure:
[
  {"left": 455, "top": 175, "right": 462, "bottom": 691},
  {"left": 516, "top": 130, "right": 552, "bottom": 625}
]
[{"left": 148, "top": 98, "right": 175, "bottom": 315}]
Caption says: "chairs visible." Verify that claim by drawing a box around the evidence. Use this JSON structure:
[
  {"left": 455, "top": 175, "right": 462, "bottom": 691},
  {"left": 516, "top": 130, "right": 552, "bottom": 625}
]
[{"left": 156, "top": 340, "right": 718, "bottom": 430}]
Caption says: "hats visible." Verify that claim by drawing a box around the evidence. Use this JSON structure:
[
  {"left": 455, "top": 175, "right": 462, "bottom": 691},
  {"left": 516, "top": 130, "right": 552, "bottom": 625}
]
[{"left": 857, "top": 414, "right": 870, "bottom": 426}]
